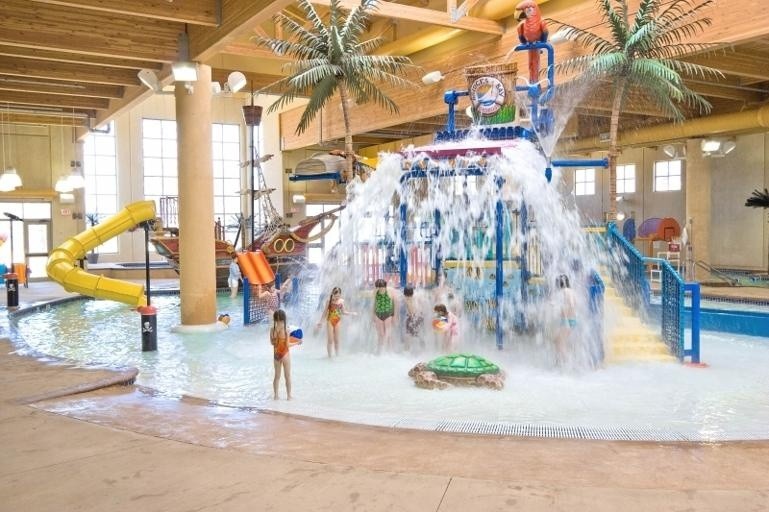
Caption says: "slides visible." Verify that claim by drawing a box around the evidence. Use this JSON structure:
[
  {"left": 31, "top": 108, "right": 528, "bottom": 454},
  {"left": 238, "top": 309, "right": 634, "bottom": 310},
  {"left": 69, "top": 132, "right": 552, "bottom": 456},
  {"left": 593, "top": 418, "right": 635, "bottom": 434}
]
[
  {"left": 235, "top": 249, "right": 275, "bottom": 285},
  {"left": 46, "top": 200, "right": 156, "bottom": 306}
]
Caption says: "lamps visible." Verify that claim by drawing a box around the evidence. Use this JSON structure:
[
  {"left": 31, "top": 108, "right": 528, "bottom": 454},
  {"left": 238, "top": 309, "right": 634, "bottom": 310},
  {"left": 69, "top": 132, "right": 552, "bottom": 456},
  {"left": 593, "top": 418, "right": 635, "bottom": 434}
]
[
  {"left": 1, "top": 88, "right": 97, "bottom": 197},
  {"left": 134, "top": 28, "right": 246, "bottom": 99},
  {"left": 660, "top": 131, "right": 743, "bottom": 161},
  {"left": 612, "top": 187, "right": 640, "bottom": 222},
  {"left": 416, "top": 69, "right": 447, "bottom": 87}
]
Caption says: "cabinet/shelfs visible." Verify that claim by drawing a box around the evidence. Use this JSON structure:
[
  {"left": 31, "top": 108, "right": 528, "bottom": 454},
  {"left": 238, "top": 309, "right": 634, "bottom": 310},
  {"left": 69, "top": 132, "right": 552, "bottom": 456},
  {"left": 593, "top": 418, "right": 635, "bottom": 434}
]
[{"left": 651, "top": 242, "right": 684, "bottom": 287}]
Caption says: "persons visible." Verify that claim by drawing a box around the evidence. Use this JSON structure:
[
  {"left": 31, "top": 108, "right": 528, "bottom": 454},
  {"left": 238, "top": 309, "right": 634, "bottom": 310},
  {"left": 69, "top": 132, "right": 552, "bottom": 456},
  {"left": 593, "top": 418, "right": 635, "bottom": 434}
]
[
  {"left": 369, "top": 278, "right": 399, "bottom": 355},
  {"left": 554, "top": 273, "right": 579, "bottom": 359},
  {"left": 227, "top": 253, "right": 243, "bottom": 297},
  {"left": 269, "top": 310, "right": 294, "bottom": 401},
  {"left": 402, "top": 284, "right": 426, "bottom": 348},
  {"left": 447, "top": 294, "right": 461, "bottom": 317},
  {"left": 430, "top": 271, "right": 450, "bottom": 304},
  {"left": 317, "top": 287, "right": 359, "bottom": 358},
  {"left": 433, "top": 304, "right": 459, "bottom": 347},
  {"left": 257, "top": 282, "right": 287, "bottom": 319}
]
